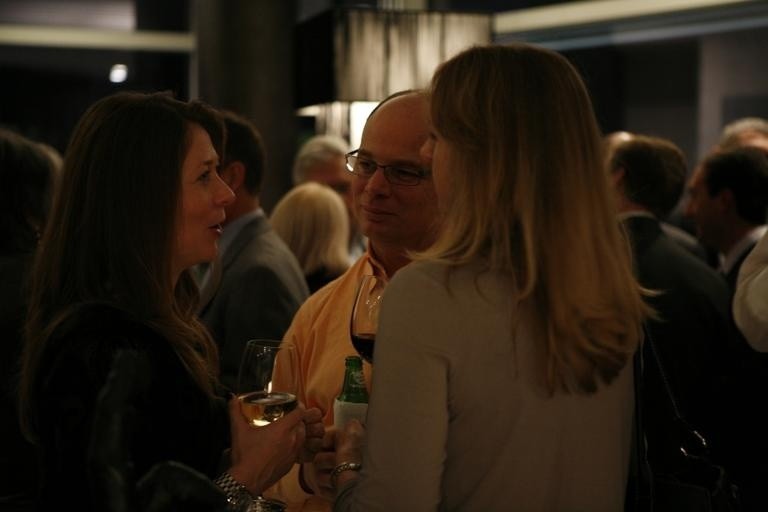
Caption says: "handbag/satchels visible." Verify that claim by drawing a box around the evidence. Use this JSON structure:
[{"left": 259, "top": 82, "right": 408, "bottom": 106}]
[{"left": 624, "top": 317, "right": 741, "bottom": 511}]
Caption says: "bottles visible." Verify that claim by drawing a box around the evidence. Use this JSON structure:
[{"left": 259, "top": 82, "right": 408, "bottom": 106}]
[{"left": 332, "top": 354, "right": 370, "bottom": 429}]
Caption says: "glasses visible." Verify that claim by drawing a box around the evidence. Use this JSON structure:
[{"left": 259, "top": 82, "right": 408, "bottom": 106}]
[{"left": 343, "top": 149, "right": 432, "bottom": 186}]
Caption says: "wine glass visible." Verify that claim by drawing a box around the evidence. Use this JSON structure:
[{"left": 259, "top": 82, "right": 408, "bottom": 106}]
[{"left": 236, "top": 339, "right": 308, "bottom": 512}]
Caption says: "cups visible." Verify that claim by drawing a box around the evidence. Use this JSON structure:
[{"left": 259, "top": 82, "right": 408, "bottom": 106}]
[{"left": 349, "top": 273, "right": 384, "bottom": 358}]
